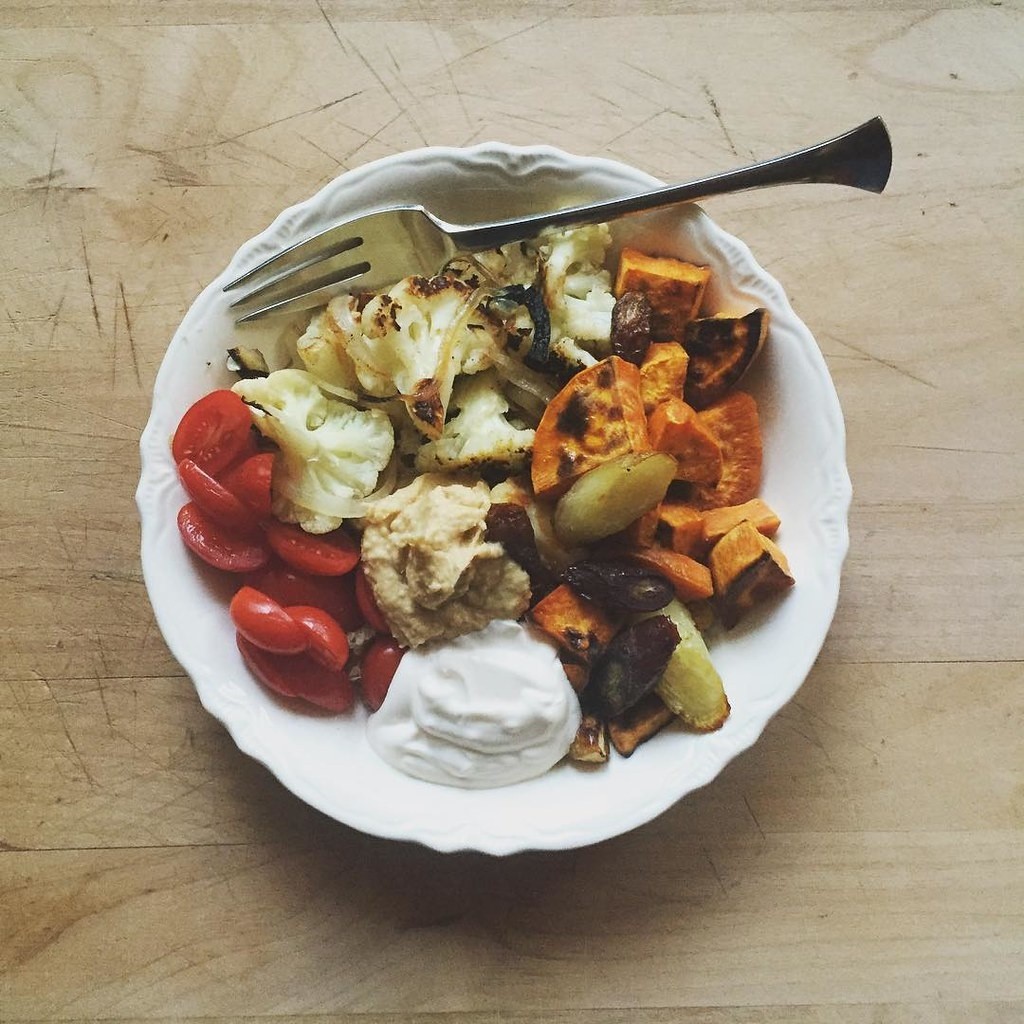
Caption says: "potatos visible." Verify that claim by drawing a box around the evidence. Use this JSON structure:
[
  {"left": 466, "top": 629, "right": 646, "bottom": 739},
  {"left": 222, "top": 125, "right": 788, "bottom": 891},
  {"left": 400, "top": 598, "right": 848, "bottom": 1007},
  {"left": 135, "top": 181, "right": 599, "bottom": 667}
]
[{"left": 528, "top": 251, "right": 796, "bottom": 775}]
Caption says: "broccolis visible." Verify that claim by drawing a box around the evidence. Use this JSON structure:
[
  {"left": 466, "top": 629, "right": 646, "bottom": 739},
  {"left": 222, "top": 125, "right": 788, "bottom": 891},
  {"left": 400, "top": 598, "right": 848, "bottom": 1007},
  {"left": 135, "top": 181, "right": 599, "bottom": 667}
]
[{"left": 228, "top": 214, "right": 622, "bottom": 532}]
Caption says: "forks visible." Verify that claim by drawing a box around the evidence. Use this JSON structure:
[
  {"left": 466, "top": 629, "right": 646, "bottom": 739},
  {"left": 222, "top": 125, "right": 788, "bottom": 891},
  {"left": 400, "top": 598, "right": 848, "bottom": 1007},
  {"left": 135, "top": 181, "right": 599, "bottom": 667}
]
[{"left": 223, "top": 113, "right": 894, "bottom": 328}]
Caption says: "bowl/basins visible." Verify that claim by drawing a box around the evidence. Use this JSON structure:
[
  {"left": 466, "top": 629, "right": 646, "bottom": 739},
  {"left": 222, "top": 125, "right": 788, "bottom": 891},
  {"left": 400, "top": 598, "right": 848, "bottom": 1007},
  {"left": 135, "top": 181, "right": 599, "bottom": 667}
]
[{"left": 134, "top": 142, "right": 856, "bottom": 859}]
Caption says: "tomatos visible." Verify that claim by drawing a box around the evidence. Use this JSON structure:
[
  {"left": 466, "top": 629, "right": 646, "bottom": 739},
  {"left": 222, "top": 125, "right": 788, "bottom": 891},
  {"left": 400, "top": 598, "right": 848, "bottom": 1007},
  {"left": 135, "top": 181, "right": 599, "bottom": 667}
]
[{"left": 170, "top": 389, "right": 403, "bottom": 717}]
